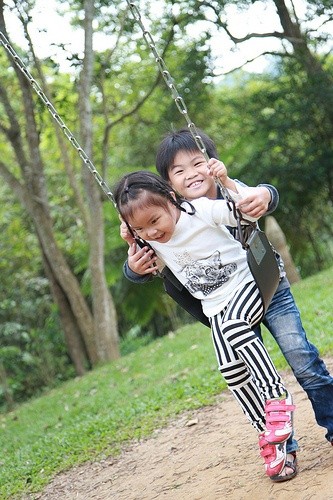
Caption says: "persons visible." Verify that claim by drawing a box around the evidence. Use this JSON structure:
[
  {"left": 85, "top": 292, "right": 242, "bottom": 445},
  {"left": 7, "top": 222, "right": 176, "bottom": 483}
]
[
  {"left": 114, "top": 159, "right": 296, "bottom": 479},
  {"left": 122, "top": 127, "right": 333, "bottom": 483}
]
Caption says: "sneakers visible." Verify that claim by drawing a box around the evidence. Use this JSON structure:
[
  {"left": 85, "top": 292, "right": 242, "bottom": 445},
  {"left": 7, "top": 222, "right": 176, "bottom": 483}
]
[
  {"left": 263, "top": 389, "right": 296, "bottom": 444},
  {"left": 258, "top": 430, "right": 286, "bottom": 477}
]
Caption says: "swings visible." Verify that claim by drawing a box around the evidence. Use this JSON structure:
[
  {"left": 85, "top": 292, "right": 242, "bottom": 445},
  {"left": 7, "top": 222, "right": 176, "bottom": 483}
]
[{"left": 0, "top": 0, "right": 280, "bottom": 330}]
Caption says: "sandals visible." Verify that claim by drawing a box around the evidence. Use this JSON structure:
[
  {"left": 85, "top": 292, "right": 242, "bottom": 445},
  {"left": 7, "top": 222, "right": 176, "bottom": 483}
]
[{"left": 271, "top": 451, "right": 298, "bottom": 482}]
